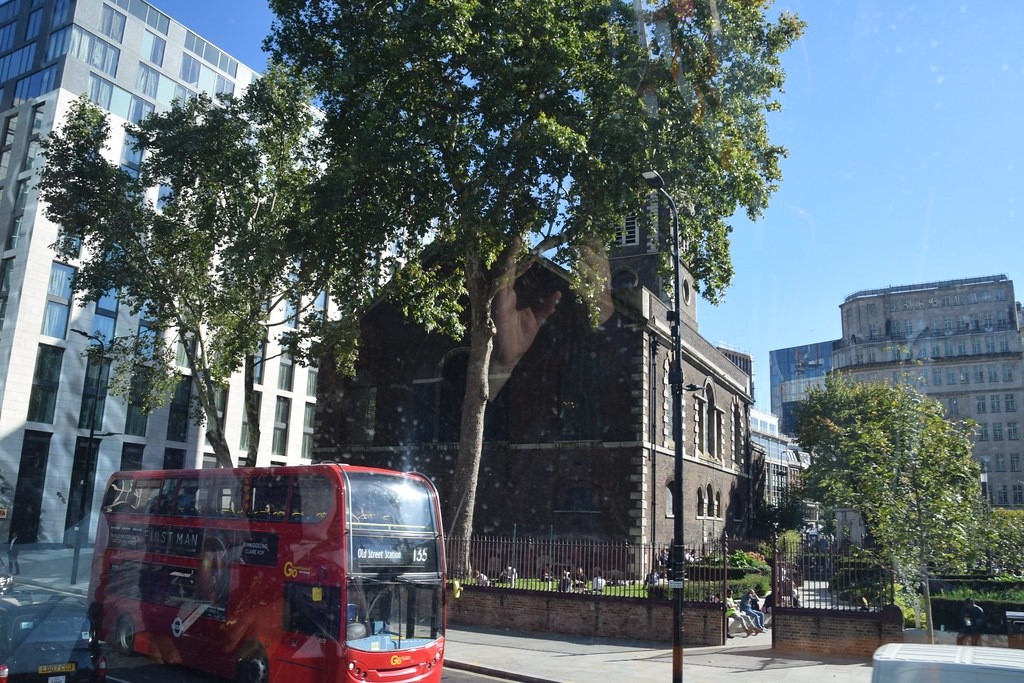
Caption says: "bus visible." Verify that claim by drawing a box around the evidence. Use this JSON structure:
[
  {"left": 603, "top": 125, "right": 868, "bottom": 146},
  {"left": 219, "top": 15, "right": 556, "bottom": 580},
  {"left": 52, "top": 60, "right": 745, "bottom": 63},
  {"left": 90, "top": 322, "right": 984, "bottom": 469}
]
[{"left": 88, "top": 461, "right": 465, "bottom": 683}]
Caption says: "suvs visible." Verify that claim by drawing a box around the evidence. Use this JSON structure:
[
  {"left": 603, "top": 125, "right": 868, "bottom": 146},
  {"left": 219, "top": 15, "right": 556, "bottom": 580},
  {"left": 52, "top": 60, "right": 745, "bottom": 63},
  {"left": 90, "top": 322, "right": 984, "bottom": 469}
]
[{"left": 0, "top": 587, "right": 109, "bottom": 683}]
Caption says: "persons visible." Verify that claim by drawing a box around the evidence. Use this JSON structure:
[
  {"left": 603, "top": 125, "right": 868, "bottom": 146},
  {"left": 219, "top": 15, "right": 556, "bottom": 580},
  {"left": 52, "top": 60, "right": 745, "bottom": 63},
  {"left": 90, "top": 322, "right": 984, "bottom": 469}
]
[
  {"left": 264, "top": 504, "right": 276, "bottom": 514},
  {"left": 642, "top": 548, "right": 697, "bottom": 592},
  {"left": 147, "top": 486, "right": 196, "bottom": 514},
  {"left": 8, "top": 532, "right": 20, "bottom": 575},
  {"left": 702, "top": 568, "right": 803, "bottom": 638},
  {"left": 794, "top": 536, "right": 833, "bottom": 581},
  {"left": 291, "top": 595, "right": 334, "bottom": 637},
  {"left": 475, "top": 566, "right": 606, "bottom": 595},
  {"left": 857, "top": 595, "right": 878, "bottom": 613},
  {"left": 957, "top": 598, "right": 985, "bottom": 646},
  {"left": 989, "top": 565, "right": 1024, "bottom": 579}
]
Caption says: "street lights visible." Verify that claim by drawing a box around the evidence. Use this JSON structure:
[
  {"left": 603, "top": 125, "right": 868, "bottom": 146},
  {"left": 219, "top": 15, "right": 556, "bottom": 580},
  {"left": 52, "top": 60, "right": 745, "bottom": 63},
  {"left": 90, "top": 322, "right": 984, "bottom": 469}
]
[
  {"left": 778, "top": 449, "right": 787, "bottom": 513},
  {"left": 641, "top": 169, "right": 688, "bottom": 683},
  {"left": 69, "top": 325, "right": 106, "bottom": 585}
]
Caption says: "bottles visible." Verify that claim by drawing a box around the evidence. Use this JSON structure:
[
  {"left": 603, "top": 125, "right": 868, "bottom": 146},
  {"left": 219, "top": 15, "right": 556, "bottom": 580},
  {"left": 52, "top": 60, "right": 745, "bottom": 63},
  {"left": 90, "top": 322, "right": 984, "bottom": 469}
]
[{"left": 763, "top": 606, "right": 766, "bottom": 614}]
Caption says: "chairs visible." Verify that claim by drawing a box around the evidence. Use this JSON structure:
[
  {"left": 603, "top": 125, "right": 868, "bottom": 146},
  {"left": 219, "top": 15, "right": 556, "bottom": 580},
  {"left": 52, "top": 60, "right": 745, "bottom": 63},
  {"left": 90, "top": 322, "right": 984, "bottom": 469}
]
[
  {"left": 129, "top": 501, "right": 322, "bottom": 522},
  {"left": 348, "top": 602, "right": 357, "bottom": 622}
]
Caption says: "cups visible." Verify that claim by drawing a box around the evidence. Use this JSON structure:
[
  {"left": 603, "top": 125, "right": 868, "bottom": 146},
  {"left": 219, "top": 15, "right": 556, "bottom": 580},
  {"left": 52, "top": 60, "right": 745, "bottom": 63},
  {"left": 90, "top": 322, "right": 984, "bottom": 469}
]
[{"left": 941, "top": 625, "right": 945, "bottom": 632}]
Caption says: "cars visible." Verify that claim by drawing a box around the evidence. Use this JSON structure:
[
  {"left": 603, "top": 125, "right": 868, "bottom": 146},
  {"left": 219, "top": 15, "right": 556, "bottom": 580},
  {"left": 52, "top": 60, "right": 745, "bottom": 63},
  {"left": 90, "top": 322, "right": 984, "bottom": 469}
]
[{"left": 0, "top": 556, "right": 15, "bottom": 596}]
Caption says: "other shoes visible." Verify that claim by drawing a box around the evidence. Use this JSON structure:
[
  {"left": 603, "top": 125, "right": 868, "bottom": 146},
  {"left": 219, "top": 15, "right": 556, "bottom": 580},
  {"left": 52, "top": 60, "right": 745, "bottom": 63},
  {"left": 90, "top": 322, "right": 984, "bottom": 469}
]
[
  {"left": 755, "top": 628, "right": 762, "bottom": 634},
  {"left": 746, "top": 629, "right": 753, "bottom": 635},
  {"left": 727, "top": 634, "right": 733, "bottom": 638}
]
